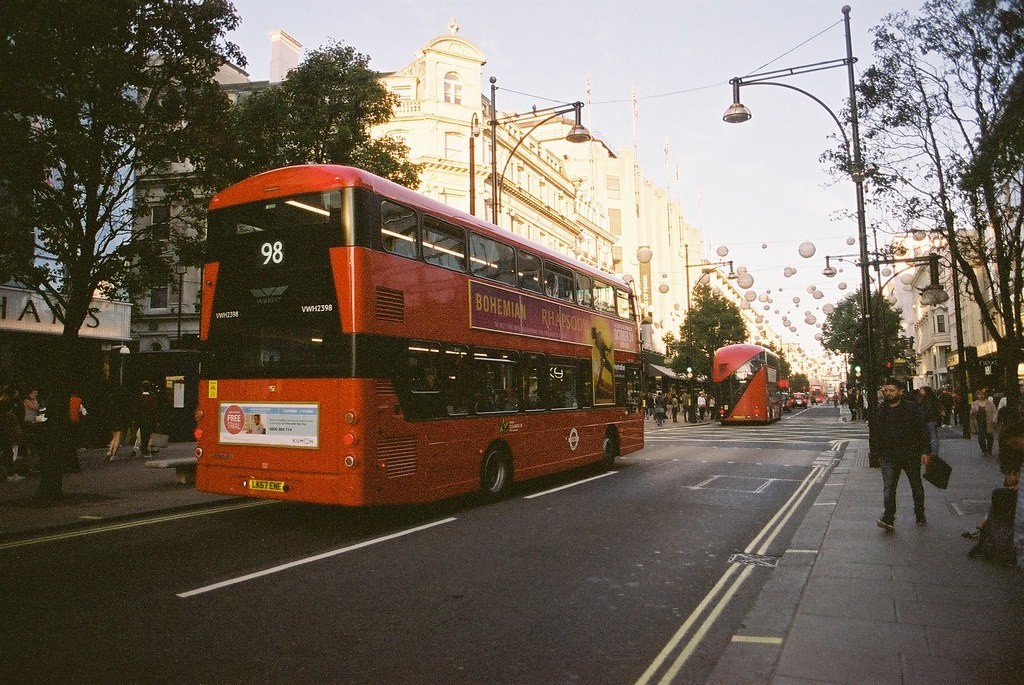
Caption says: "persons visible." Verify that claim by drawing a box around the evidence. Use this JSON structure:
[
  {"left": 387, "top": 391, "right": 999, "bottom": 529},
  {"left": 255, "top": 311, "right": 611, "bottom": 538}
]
[
  {"left": 833, "top": 386, "right": 1024, "bottom": 456},
  {"left": 249, "top": 413, "right": 263, "bottom": 434},
  {"left": 644, "top": 388, "right": 715, "bottom": 427},
  {"left": 872, "top": 379, "right": 931, "bottom": 529},
  {"left": 592, "top": 329, "right": 615, "bottom": 403},
  {"left": 961, "top": 402, "right": 1024, "bottom": 568},
  {"left": 0, "top": 378, "right": 159, "bottom": 480},
  {"left": 810, "top": 391, "right": 830, "bottom": 403}
]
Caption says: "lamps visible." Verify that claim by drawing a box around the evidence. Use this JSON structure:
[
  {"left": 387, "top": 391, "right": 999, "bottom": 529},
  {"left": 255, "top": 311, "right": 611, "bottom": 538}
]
[{"left": 112, "top": 345, "right": 131, "bottom": 354}]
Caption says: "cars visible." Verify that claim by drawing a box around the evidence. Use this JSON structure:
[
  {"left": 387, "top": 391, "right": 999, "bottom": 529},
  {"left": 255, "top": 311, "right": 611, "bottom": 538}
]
[
  {"left": 781, "top": 393, "right": 795, "bottom": 412},
  {"left": 794, "top": 393, "right": 807, "bottom": 407},
  {"left": 814, "top": 396, "right": 823, "bottom": 403}
]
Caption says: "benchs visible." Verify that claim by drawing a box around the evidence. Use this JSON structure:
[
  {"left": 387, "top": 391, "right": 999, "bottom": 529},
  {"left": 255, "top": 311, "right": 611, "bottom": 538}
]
[{"left": 145, "top": 457, "right": 197, "bottom": 484}]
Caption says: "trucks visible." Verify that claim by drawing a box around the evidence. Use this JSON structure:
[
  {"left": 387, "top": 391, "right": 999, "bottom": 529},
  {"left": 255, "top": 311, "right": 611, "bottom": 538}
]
[
  {"left": 825, "top": 387, "right": 836, "bottom": 401},
  {"left": 810, "top": 385, "right": 823, "bottom": 399}
]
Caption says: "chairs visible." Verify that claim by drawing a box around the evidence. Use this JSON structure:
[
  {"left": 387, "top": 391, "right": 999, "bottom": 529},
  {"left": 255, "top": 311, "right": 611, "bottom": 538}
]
[
  {"left": 423, "top": 241, "right": 462, "bottom": 270},
  {"left": 475, "top": 393, "right": 496, "bottom": 413},
  {"left": 492, "top": 270, "right": 515, "bottom": 285},
  {"left": 383, "top": 235, "right": 416, "bottom": 258},
  {"left": 547, "top": 273, "right": 559, "bottom": 297},
  {"left": 522, "top": 279, "right": 541, "bottom": 291}
]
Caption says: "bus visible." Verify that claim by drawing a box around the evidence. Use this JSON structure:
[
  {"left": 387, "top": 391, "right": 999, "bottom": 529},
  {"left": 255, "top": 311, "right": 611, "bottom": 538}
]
[
  {"left": 109, "top": 350, "right": 198, "bottom": 407},
  {"left": 195, "top": 164, "right": 644, "bottom": 508},
  {"left": 713, "top": 344, "right": 782, "bottom": 425}
]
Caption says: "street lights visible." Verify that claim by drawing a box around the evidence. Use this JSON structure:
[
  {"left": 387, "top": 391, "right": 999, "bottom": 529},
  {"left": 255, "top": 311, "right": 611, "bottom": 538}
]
[
  {"left": 723, "top": 6, "right": 880, "bottom": 469},
  {"left": 684, "top": 244, "right": 738, "bottom": 423},
  {"left": 172, "top": 257, "right": 188, "bottom": 349},
  {"left": 823, "top": 253, "right": 891, "bottom": 382},
  {"left": 908, "top": 228, "right": 972, "bottom": 439},
  {"left": 490, "top": 77, "right": 591, "bottom": 226},
  {"left": 469, "top": 112, "right": 480, "bottom": 216}
]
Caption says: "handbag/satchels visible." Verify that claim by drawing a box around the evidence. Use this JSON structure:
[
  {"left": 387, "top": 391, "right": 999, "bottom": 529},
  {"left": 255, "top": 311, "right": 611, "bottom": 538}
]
[{"left": 922, "top": 456, "right": 952, "bottom": 489}]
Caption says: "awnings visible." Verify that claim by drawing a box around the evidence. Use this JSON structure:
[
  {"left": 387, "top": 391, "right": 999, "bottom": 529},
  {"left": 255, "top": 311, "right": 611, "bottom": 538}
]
[{"left": 648, "top": 362, "right": 712, "bottom": 383}]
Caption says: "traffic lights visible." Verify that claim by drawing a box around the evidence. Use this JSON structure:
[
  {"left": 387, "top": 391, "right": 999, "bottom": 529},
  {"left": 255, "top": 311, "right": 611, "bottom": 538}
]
[
  {"left": 886, "top": 361, "right": 892, "bottom": 375},
  {"left": 686, "top": 356, "right": 693, "bottom": 378},
  {"left": 855, "top": 365, "right": 862, "bottom": 385},
  {"left": 966, "top": 346, "right": 980, "bottom": 371}
]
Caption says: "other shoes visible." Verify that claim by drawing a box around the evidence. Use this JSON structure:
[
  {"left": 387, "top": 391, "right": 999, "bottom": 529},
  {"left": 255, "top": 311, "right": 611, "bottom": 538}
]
[
  {"left": 916, "top": 516, "right": 928, "bottom": 526},
  {"left": 141, "top": 453, "right": 153, "bottom": 458},
  {"left": 7, "top": 474, "right": 26, "bottom": 482},
  {"left": 105, "top": 452, "right": 120, "bottom": 461},
  {"left": 961, "top": 526, "right": 983, "bottom": 541},
  {"left": 132, "top": 448, "right": 138, "bottom": 458},
  {"left": 877, "top": 514, "right": 896, "bottom": 532}
]
